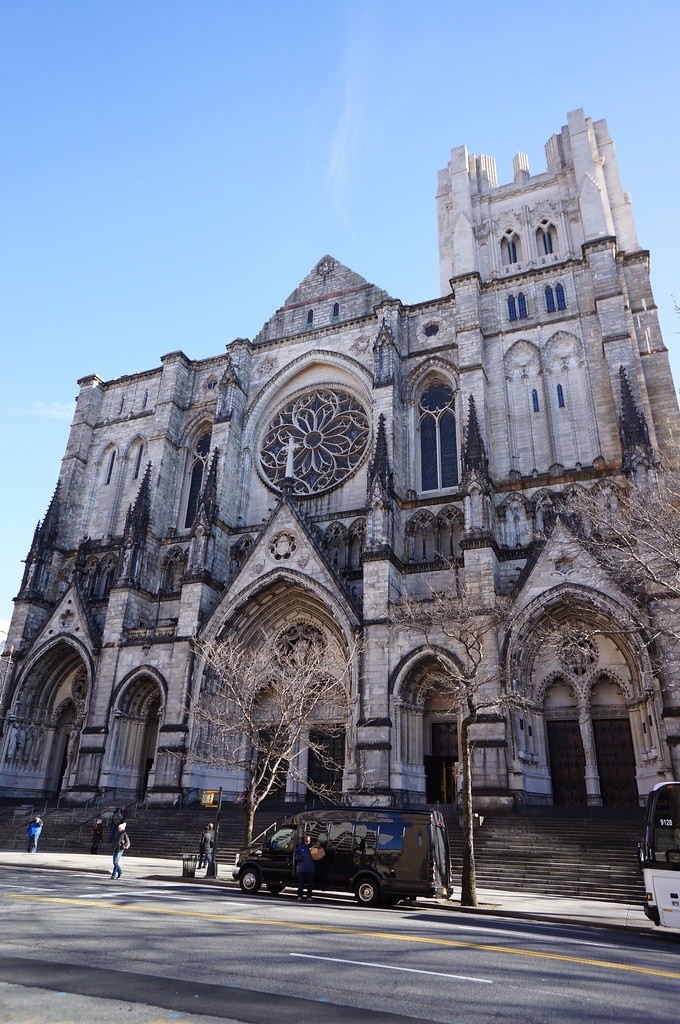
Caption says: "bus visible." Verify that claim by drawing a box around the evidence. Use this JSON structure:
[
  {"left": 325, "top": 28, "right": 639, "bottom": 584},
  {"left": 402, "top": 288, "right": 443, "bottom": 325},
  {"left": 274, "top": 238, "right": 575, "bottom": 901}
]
[{"left": 637, "top": 781, "right": 680, "bottom": 929}]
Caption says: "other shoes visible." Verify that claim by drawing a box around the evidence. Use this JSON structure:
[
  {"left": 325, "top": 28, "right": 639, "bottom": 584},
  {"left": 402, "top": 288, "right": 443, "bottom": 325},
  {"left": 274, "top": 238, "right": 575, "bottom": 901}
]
[
  {"left": 296, "top": 897, "right": 302, "bottom": 902},
  {"left": 197, "top": 866, "right": 202, "bottom": 869},
  {"left": 117, "top": 872, "right": 123, "bottom": 879},
  {"left": 202, "top": 866, "right": 206, "bottom": 868},
  {"left": 307, "top": 896, "right": 313, "bottom": 902}
]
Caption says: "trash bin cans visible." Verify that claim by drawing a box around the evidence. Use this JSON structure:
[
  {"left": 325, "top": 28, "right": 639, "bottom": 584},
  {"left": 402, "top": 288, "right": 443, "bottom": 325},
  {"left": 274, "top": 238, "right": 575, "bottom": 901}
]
[{"left": 183, "top": 854, "right": 198, "bottom": 878}]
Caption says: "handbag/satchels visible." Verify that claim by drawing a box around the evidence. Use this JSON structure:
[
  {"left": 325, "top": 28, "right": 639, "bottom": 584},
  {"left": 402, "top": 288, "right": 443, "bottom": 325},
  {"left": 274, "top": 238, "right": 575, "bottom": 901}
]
[
  {"left": 113, "top": 816, "right": 120, "bottom": 825},
  {"left": 122, "top": 838, "right": 131, "bottom": 850},
  {"left": 210, "top": 840, "right": 214, "bottom": 848}
]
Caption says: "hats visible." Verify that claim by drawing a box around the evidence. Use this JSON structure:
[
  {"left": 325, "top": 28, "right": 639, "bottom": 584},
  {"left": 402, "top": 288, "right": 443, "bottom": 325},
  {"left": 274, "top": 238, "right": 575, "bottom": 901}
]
[
  {"left": 204, "top": 826, "right": 209, "bottom": 830},
  {"left": 118, "top": 823, "right": 127, "bottom": 832}
]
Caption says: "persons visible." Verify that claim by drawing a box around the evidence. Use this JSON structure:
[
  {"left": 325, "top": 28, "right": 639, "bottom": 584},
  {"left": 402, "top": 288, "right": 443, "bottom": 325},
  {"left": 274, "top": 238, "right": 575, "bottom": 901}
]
[
  {"left": 91, "top": 819, "right": 103, "bottom": 853},
  {"left": 109, "top": 807, "right": 126, "bottom": 843},
  {"left": 8, "top": 724, "right": 45, "bottom": 760},
  {"left": 111, "top": 822, "right": 130, "bottom": 880},
  {"left": 242, "top": 787, "right": 249, "bottom": 806},
  {"left": 295, "top": 836, "right": 320, "bottom": 902},
  {"left": 26, "top": 815, "right": 43, "bottom": 853},
  {"left": 197, "top": 823, "right": 215, "bottom": 869},
  {"left": 67, "top": 726, "right": 77, "bottom": 756}
]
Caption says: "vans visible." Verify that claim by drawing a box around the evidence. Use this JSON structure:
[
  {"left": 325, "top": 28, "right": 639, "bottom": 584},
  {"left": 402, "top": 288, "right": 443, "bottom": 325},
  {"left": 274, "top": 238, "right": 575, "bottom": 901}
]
[{"left": 232, "top": 806, "right": 454, "bottom": 907}]
